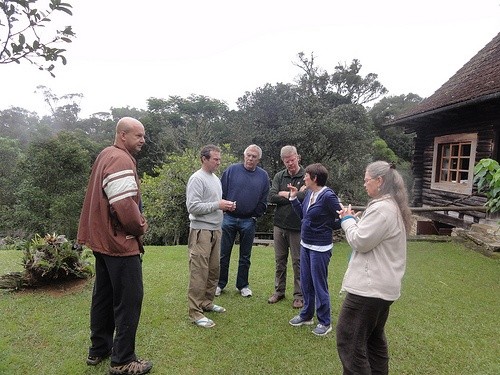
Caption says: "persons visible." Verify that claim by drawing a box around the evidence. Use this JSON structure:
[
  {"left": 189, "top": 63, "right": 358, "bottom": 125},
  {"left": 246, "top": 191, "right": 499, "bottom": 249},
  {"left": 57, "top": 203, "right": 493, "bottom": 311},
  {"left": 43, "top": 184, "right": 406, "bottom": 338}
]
[
  {"left": 186, "top": 144, "right": 237, "bottom": 327},
  {"left": 267, "top": 145, "right": 307, "bottom": 308},
  {"left": 287, "top": 162, "right": 342, "bottom": 337},
  {"left": 213, "top": 144, "right": 271, "bottom": 295},
  {"left": 336, "top": 160, "right": 413, "bottom": 375},
  {"left": 77, "top": 116, "right": 154, "bottom": 375}
]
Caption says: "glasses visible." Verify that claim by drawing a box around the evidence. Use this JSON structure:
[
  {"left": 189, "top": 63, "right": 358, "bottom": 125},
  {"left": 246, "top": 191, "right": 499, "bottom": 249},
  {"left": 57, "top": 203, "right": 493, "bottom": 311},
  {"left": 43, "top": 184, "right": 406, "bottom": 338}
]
[{"left": 363, "top": 178, "right": 372, "bottom": 183}]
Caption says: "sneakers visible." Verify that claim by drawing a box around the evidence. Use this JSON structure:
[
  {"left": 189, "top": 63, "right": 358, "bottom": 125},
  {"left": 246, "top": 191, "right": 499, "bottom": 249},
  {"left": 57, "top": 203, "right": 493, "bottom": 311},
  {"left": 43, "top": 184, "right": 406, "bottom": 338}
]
[
  {"left": 312, "top": 323, "right": 332, "bottom": 336},
  {"left": 86, "top": 346, "right": 113, "bottom": 366},
  {"left": 214, "top": 286, "right": 223, "bottom": 296},
  {"left": 236, "top": 286, "right": 252, "bottom": 297},
  {"left": 109, "top": 359, "right": 153, "bottom": 375},
  {"left": 289, "top": 315, "right": 314, "bottom": 326}
]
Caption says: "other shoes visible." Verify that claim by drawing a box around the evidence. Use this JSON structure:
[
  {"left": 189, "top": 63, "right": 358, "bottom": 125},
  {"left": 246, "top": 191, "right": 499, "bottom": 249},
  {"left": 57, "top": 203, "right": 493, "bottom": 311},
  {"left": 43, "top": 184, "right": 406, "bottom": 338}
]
[
  {"left": 208, "top": 305, "right": 226, "bottom": 313},
  {"left": 192, "top": 316, "right": 216, "bottom": 327},
  {"left": 293, "top": 299, "right": 303, "bottom": 308},
  {"left": 268, "top": 294, "right": 285, "bottom": 304}
]
